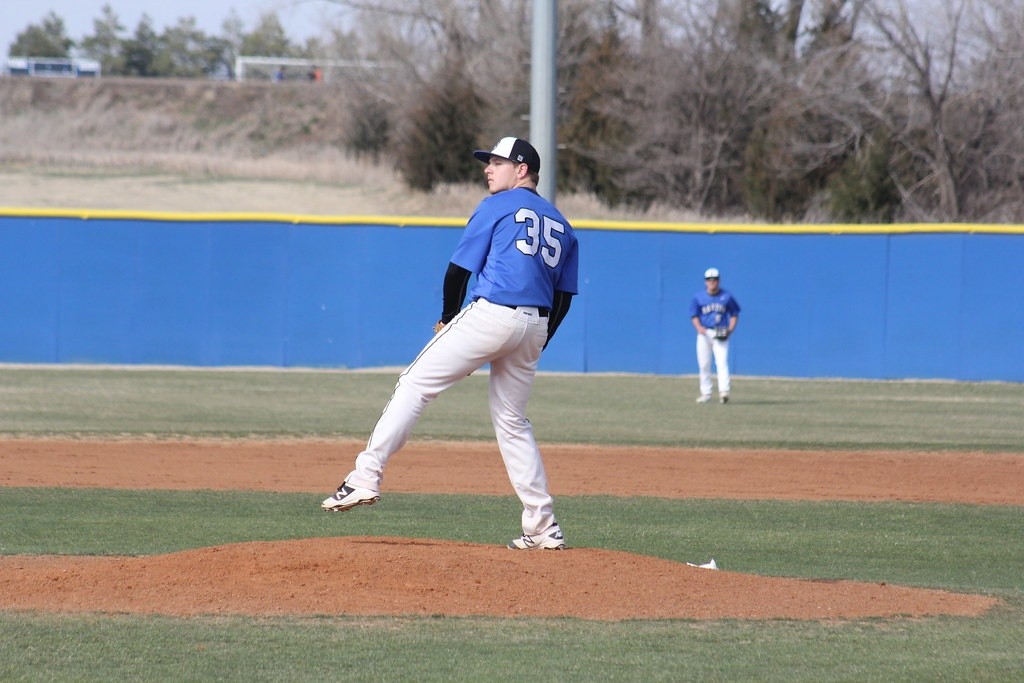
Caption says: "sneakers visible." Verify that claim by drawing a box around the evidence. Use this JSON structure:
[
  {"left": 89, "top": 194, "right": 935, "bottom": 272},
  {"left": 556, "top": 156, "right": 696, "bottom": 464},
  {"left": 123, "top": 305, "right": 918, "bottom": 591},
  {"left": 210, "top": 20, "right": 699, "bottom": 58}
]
[
  {"left": 321, "top": 480, "right": 381, "bottom": 513},
  {"left": 505, "top": 521, "right": 565, "bottom": 552}
]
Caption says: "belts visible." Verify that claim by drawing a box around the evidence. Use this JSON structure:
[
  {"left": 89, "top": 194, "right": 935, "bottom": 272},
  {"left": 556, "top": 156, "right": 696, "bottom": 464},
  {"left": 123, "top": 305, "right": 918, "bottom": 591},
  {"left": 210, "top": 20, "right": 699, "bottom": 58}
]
[{"left": 506, "top": 303, "right": 548, "bottom": 318}]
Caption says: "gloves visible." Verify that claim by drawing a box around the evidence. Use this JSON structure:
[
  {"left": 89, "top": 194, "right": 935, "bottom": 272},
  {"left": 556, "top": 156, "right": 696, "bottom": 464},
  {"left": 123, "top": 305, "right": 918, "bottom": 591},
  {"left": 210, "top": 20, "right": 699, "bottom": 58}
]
[{"left": 706, "top": 328, "right": 717, "bottom": 339}]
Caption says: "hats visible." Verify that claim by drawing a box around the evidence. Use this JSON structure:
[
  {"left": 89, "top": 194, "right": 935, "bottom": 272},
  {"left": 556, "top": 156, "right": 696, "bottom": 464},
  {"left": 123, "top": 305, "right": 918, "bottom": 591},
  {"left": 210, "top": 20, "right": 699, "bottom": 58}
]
[
  {"left": 472, "top": 136, "right": 540, "bottom": 175},
  {"left": 704, "top": 268, "right": 720, "bottom": 280}
]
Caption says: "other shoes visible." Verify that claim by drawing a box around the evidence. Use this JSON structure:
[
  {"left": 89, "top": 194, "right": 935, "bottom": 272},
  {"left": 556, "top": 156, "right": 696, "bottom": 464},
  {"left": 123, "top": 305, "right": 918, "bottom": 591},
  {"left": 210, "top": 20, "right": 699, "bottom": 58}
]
[
  {"left": 720, "top": 396, "right": 730, "bottom": 404},
  {"left": 696, "top": 395, "right": 713, "bottom": 403}
]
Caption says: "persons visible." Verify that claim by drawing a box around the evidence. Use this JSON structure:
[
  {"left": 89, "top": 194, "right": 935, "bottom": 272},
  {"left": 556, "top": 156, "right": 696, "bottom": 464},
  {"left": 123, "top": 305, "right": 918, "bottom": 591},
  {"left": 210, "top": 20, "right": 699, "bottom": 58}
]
[
  {"left": 319, "top": 135, "right": 579, "bottom": 553},
  {"left": 689, "top": 266, "right": 741, "bottom": 402},
  {"left": 308, "top": 64, "right": 321, "bottom": 80},
  {"left": 276, "top": 65, "right": 287, "bottom": 80}
]
